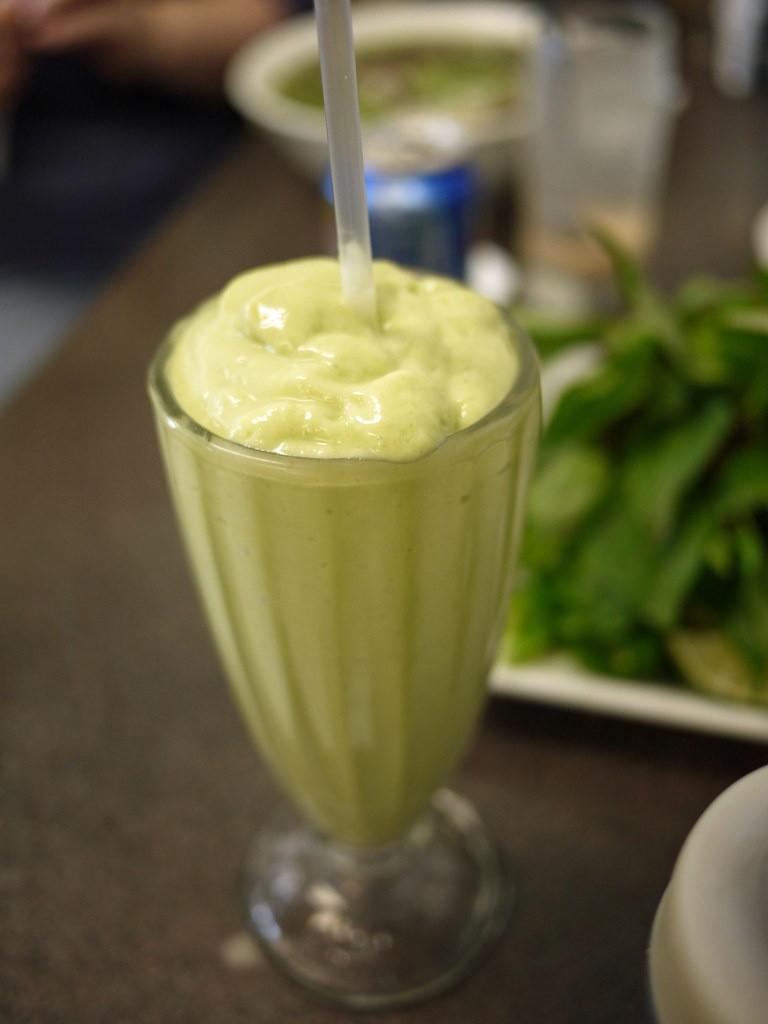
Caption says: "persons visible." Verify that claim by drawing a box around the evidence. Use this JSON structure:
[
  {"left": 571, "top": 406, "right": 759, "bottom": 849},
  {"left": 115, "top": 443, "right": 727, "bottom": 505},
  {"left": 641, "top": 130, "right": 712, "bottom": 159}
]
[{"left": 0, "top": 0, "right": 316, "bottom": 288}]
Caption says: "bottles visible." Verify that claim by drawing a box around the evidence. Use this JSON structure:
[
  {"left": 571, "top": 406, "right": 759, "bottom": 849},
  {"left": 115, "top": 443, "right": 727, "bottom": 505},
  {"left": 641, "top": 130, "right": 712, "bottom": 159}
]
[{"left": 525, "top": 14, "right": 675, "bottom": 329}]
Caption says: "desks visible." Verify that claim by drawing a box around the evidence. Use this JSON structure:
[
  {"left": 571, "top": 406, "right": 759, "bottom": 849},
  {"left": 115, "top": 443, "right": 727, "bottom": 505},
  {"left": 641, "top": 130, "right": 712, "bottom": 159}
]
[{"left": 0, "top": 41, "right": 768, "bottom": 1024}]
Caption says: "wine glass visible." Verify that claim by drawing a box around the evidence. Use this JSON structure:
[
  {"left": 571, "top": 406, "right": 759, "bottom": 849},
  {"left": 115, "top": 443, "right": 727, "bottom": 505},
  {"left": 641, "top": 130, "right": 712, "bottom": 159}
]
[{"left": 145, "top": 254, "right": 547, "bottom": 1007}]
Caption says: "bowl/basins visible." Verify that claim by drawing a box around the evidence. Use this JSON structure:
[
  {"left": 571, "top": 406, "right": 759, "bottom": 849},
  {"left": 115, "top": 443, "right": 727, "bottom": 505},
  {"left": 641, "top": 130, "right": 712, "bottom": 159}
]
[{"left": 223, "top": 0, "right": 687, "bottom": 198}]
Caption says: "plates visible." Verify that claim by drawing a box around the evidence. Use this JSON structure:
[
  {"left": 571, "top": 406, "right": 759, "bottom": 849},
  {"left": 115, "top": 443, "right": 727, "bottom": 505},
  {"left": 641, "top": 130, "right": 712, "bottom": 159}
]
[{"left": 483, "top": 338, "right": 768, "bottom": 745}]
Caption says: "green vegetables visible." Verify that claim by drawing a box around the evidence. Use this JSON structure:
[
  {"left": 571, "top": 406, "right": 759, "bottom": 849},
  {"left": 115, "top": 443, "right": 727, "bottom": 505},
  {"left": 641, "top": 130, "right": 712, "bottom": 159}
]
[{"left": 491, "top": 224, "right": 768, "bottom": 716}]
